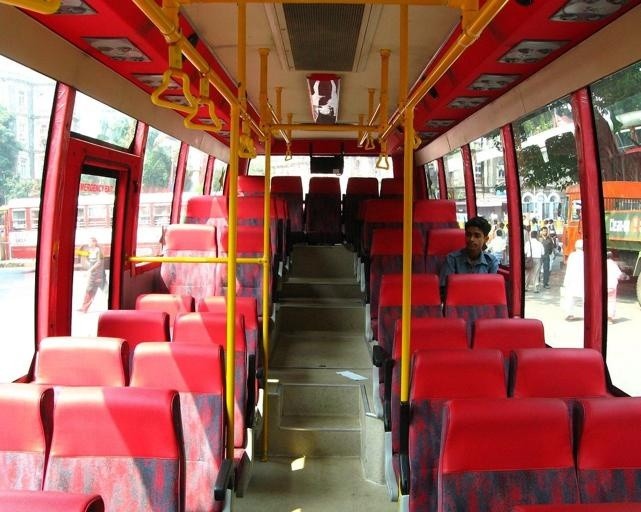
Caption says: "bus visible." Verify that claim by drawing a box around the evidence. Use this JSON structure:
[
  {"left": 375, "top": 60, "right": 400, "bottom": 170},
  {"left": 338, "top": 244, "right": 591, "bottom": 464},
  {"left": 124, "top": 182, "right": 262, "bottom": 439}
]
[{"left": 5, "top": 193, "right": 200, "bottom": 268}]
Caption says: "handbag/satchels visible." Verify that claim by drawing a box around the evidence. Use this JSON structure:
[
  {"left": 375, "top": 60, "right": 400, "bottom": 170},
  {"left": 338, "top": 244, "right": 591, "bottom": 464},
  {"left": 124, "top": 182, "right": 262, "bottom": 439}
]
[{"left": 525, "top": 257, "right": 533, "bottom": 269}]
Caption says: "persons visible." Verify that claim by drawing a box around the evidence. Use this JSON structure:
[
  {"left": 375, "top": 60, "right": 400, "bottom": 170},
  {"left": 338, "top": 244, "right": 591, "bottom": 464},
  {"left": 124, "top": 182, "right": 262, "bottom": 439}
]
[
  {"left": 485, "top": 209, "right": 558, "bottom": 294},
  {"left": 559, "top": 239, "right": 585, "bottom": 322},
  {"left": 605, "top": 251, "right": 623, "bottom": 326},
  {"left": 76, "top": 235, "right": 109, "bottom": 314},
  {"left": 438, "top": 216, "right": 501, "bottom": 311}
]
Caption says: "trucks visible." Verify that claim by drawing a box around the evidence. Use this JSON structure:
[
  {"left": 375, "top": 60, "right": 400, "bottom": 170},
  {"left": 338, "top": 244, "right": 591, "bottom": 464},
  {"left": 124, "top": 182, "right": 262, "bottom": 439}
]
[{"left": 564, "top": 181, "right": 641, "bottom": 308}]
